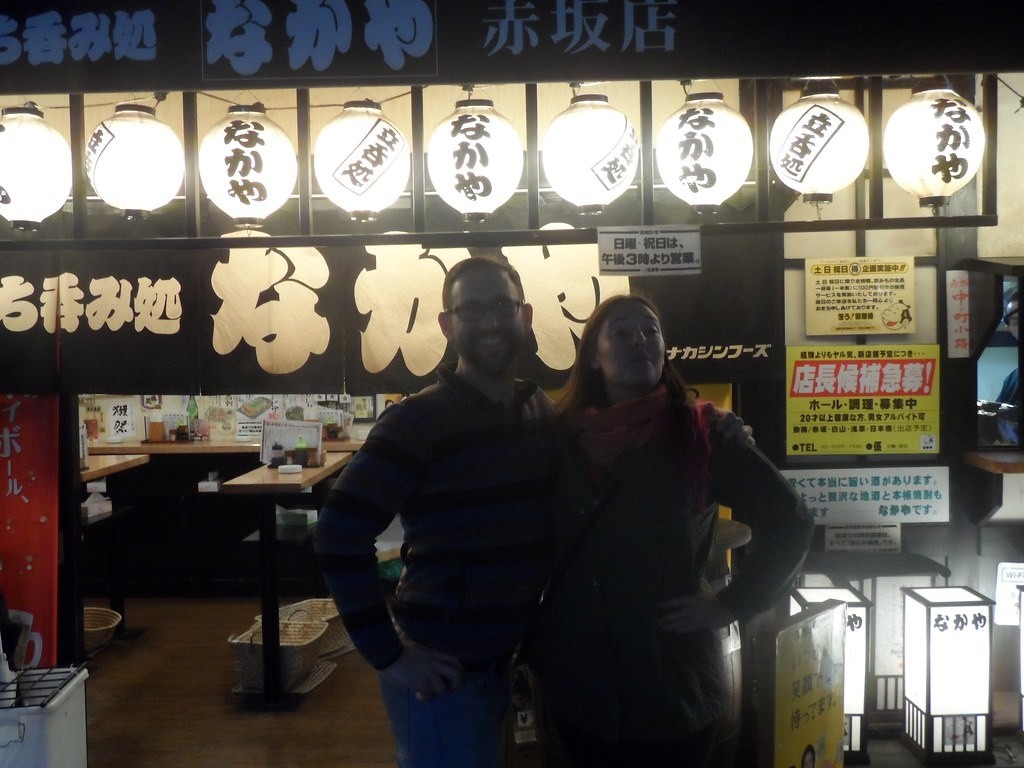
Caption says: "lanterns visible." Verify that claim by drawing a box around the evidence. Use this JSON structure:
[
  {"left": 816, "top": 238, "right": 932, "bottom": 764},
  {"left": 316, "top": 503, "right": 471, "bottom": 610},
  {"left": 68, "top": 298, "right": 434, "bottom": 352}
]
[
  {"left": 197, "top": 105, "right": 297, "bottom": 229},
  {"left": 314, "top": 101, "right": 411, "bottom": 223},
  {"left": 0, "top": 107, "right": 73, "bottom": 231},
  {"left": 769, "top": 86, "right": 870, "bottom": 202},
  {"left": 427, "top": 99, "right": 524, "bottom": 222},
  {"left": 542, "top": 94, "right": 639, "bottom": 216},
  {"left": 882, "top": 80, "right": 985, "bottom": 207},
  {"left": 85, "top": 104, "right": 185, "bottom": 222},
  {"left": 655, "top": 92, "right": 754, "bottom": 212}
]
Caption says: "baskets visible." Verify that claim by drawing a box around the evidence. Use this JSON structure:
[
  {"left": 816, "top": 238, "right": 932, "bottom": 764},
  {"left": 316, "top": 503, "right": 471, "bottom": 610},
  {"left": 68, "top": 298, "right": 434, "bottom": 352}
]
[
  {"left": 224, "top": 619, "right": 338, "bottom": 694},
  {"left": 83, "top": 607, "right": 122, "bottom": 651},
  {"left": 254, "top": 597, "right": 357, "bottom": 660}
]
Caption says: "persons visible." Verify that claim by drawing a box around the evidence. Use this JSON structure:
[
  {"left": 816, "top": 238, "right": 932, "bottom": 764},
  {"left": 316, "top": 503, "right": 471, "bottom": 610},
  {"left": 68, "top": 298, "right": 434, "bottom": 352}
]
[
  {"left": 975, "top": 285, "right": 1019, "bottom": 445},
  {"left": 401, "top": 393, "right": 410, "bottom": 401},
  {"left": 801, "top": 744, "right": 815, "bottom": 768},
  {"left": 525, "top": 295, "right": 815, "bottom": 768},
  {"left": 385, "top": 399, "right": 393, "bottom": 408},
  {"left": 314, "top": 255, "right": 754, "bottom": 768}
]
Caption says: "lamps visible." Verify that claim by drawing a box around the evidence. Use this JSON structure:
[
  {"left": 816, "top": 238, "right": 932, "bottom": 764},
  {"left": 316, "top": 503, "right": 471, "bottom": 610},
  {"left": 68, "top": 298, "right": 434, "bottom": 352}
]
[{"left": 713, "top": 553, "right": 1024, "bottom": 767}]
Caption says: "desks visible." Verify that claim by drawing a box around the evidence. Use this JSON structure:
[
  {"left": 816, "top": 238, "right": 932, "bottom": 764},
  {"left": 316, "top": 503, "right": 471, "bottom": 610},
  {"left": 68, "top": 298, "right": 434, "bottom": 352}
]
[
  {"left": 79, "top": 454, "right": 150, "bottom": 642},
  {"left": 223, "top": 443, "right": 347, "bottom": 715},
  {"left": 86, "top": 434, "right": 377, "bottom": 453}
]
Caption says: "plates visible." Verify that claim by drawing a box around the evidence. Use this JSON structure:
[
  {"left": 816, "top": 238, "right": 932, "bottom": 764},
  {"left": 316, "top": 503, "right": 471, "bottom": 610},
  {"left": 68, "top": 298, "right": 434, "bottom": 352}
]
[{"left": 236, "top": 396, "right": 272, "bottom": 419}]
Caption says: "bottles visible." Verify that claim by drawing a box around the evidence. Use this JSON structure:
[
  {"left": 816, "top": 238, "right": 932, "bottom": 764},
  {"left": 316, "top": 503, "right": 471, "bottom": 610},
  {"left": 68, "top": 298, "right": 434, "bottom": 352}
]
[
  {"left": 271, "top": 440, "right": 284, "bottom": 467},
  {"left": 186, "top": 394, "right": 198, "bottom": 440},
  {"left": 327, "top": 416, "right": 337, "bottom": 440},
  {"left": 295, "top": 437, "right": 306, "bottom": 467}
]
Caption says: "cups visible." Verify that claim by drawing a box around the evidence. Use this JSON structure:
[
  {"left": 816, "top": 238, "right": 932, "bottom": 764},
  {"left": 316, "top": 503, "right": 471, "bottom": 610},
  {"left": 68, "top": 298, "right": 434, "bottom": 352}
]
[{"left": 149, "top": 413, "right": 165, "bottom": 442}]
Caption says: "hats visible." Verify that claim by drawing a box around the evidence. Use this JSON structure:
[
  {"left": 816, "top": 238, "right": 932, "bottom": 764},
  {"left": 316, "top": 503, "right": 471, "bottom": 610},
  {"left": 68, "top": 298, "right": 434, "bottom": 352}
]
[{"left": 1003, "top": 289, "right": 1020, "bottom": 325}]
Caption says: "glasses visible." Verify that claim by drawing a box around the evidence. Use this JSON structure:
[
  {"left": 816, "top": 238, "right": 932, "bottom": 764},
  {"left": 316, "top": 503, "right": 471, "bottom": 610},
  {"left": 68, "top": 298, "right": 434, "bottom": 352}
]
[{"left": 446, "top": 298, "right": 525, "bottom": 322}]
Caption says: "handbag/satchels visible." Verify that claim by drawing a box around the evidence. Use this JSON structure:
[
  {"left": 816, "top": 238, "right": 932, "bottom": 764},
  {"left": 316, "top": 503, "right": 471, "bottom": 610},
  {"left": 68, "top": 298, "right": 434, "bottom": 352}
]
[{"left": 494, "top": 665, "right": 553, "bottom": 768}]
